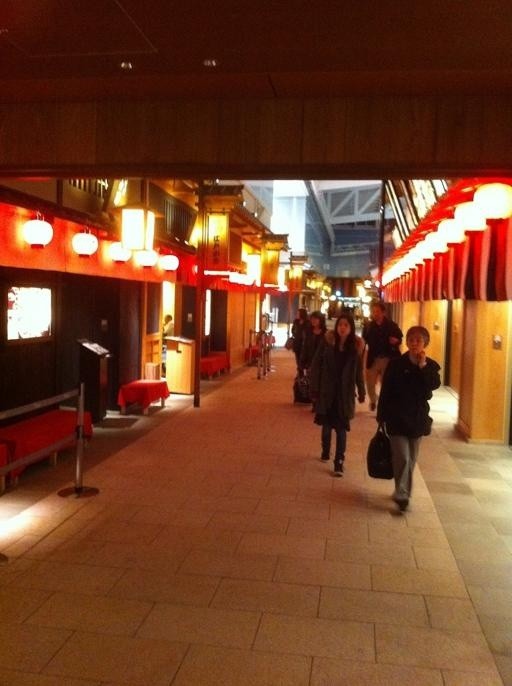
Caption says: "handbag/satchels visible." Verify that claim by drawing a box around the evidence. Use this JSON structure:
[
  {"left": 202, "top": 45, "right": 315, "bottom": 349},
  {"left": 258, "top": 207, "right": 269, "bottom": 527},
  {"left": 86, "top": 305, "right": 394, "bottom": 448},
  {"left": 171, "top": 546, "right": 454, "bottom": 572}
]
[{"left": 366, "top": 425, "right": 394, "bottom": 480}]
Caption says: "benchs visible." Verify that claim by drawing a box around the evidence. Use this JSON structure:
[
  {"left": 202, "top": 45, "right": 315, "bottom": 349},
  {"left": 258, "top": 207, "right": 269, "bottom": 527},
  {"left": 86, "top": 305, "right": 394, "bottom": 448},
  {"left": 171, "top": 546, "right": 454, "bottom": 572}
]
[
  {"left": 0, "top": 406, "right": 94, "bottom": 495},
  {"left": 245, "top": 343, "right": 262, "bottom": 366},
  {"left": 199, "top": 348, "right": 229, "bottom": 382},
  {"left": 115, "top": 377, "right": 171, "bottom": 417}
]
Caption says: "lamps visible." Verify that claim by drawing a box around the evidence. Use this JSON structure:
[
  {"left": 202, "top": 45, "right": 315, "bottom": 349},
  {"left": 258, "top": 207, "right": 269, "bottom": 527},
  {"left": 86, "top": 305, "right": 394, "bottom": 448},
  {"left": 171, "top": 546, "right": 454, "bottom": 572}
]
[{"left": 18, "top": 209, "right": 182, "bottom": 273}]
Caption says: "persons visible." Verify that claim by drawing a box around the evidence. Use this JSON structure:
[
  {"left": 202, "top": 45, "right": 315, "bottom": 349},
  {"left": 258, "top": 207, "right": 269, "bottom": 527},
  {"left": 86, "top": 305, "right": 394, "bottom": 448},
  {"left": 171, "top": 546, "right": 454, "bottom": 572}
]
[
  {"left": 161, "top": 314, "right": 174, "bottom": 335},
  {"left": 375, "top": 325, "right": 441, "bottom": 518},
  {"left": 359, "top": 301, "right": 403, "bottom": 414},
  {"left": 291, "top": 308, "right": 310, "bottom": 362},
  {"left": 298, "top": 310, "right": 327, "bottom": 412},
  {"left": 308, "top": 313, "right": 366, "bottom": 479}
]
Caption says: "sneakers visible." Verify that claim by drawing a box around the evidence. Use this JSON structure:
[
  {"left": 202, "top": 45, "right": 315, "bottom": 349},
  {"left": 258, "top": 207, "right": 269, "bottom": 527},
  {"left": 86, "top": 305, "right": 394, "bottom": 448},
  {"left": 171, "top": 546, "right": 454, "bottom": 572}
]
[
  {"left": 334, "top": 460, "right": 343, "bottom": 476},
  {"left": 395, "top": 498, "right": 410, "bottom": 511},
  {"left": 371, "top": 403, "right": 377, "bottom": 411},
  {"left": 321, "top": 450, "right": 329, "bottom": 462}
]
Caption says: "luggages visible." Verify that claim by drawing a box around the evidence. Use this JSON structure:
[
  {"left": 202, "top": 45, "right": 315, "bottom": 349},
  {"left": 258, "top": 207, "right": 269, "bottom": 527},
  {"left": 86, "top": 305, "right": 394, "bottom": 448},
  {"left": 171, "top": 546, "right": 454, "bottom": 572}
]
[{"left": 294, "top": 367, "right": 313, "bottom": 404}]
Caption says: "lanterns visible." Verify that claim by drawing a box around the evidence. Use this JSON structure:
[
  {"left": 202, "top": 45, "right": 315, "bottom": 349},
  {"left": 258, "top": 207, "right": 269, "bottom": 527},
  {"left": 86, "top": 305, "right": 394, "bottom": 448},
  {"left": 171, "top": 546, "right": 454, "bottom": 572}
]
[
  {"left": 370, "top": 182, "right": 512, "bottom": 291},
  {"left": 19, "top": 218, "right": 256, "bottom": 285}
]
